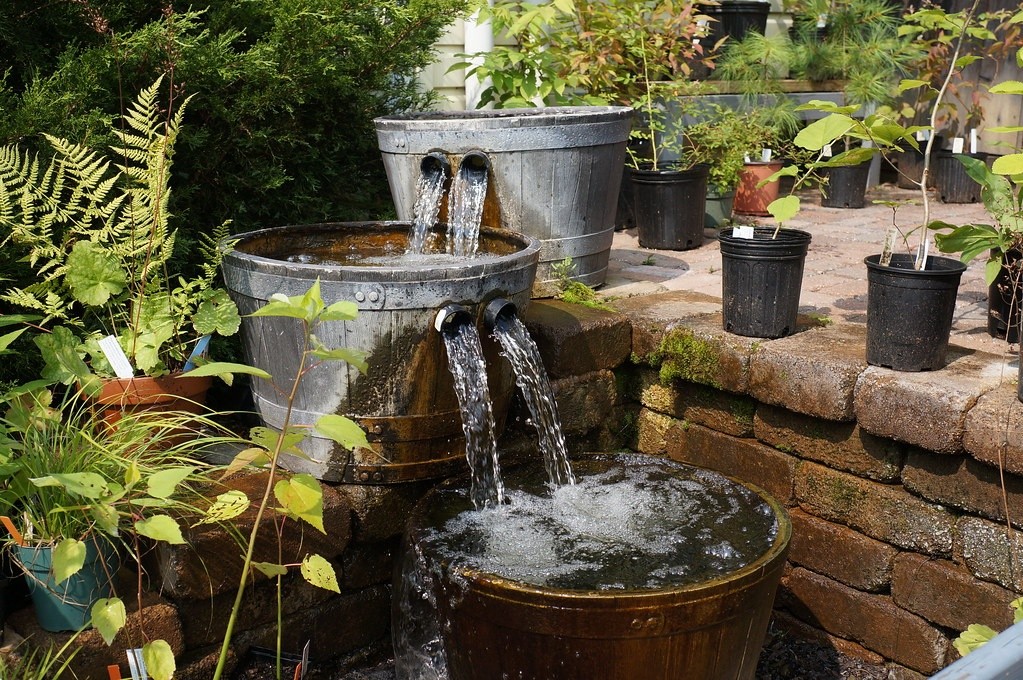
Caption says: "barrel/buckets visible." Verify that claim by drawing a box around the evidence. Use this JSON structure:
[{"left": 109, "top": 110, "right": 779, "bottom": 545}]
[
  {"left": 374, "top": 106, "right": 633, "bottom": 296},
  {"left": 219, "top": 221, "right": 540, "bottom": 485},
  {"left": 408, "top": 452, "right": 791, "bottom": 680}
]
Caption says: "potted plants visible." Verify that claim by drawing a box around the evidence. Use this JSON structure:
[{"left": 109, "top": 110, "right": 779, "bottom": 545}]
[
  {"left": 791, "top": 0, "right": 1023, "bottom": 373},
  {"left": 558, "top": 0, "right": 753, "bottom": 250},
  {"left": 0, "top": 71, "right": 244, "bottom": 464},
  {"left": 658, "top": 0, "right": 1023, "bottom": 216},
  {"left": 927, "top": 50, "right": 1023, "bottom": 343},
  {"left": 719, "top": 98, "right": 925, "bottom": 338},
  {"left": 0, "top": 368, "right": 300, "bottom": 633},
  {"left": 449, "top": 0, "right": 729, "bottom": 232}
]
